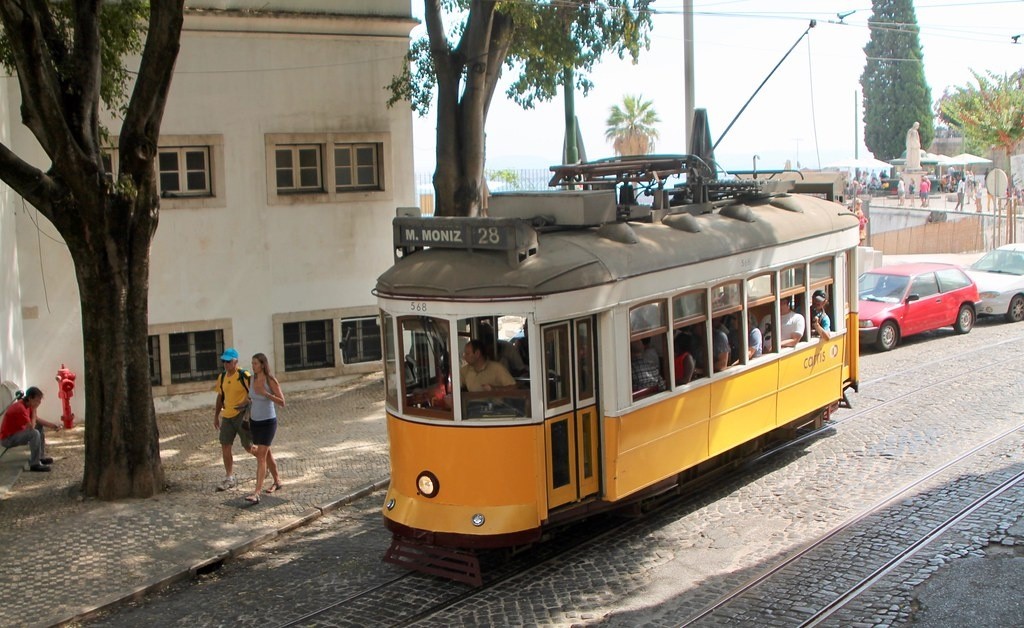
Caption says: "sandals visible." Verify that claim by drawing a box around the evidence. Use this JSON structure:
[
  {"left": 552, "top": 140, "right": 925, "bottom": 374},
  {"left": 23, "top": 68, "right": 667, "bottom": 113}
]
[
  {"left": 266, "top": 479, "right": 284, "bottom": 493},
  {"left": 245, "top": 492, "right": 261, "bottom": 503}
]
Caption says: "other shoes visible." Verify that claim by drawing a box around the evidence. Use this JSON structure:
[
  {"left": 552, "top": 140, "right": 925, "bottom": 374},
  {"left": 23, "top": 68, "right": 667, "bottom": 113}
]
[{"left": 30, "top": 457, "right": 53, "bottom": 471}]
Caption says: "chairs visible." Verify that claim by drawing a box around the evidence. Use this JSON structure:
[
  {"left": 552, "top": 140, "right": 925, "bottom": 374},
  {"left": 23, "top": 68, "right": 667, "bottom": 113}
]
[{"left": 496, "top": 338, "right": 531, "bottom": 384}]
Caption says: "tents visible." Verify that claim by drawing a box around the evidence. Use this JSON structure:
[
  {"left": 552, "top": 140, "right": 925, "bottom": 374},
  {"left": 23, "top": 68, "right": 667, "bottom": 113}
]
[{"left": 924, "top": 152, "right": 994, "bottom": 187}]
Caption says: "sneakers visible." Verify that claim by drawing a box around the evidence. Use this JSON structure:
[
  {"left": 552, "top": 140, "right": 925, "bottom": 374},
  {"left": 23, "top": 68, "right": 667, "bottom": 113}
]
[{"left": 217, "top": 478, "right": 235, "bottom": 491}]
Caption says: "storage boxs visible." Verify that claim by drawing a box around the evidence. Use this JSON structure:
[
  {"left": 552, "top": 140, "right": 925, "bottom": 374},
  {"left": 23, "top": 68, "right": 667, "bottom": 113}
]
[{"left": 488, "top": 187, "right": 616, "bottom": 226}]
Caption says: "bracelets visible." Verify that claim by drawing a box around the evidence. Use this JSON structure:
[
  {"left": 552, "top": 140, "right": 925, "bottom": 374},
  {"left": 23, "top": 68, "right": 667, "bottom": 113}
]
[{"left": 265, "top": 392, "right": 271, "bottom": 399}]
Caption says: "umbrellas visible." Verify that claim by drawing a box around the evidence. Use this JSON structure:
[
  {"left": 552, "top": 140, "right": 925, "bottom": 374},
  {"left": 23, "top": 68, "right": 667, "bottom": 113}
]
[
  {"left": 562, "top": 115, "right": 588, "bottom": 172},
  {"left": 688, "top": 106, "right": 720, "bottom": 185}
]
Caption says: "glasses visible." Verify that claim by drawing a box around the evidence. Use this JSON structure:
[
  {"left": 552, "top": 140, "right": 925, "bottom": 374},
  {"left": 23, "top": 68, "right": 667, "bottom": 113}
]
[{"left": 221, "top": 360, "right": 231, "bottom": 363}]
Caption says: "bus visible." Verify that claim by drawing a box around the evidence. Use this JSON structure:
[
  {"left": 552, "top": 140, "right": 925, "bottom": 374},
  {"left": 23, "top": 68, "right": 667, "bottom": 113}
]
[{"left": 369, "top": 154, "right": 861, "bottom": 587}]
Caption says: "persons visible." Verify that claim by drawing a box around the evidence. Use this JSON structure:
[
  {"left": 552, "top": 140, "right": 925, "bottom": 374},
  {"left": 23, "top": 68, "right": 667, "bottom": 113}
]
[
  {"left": 905, "top": 121, "right": 923, "bottom": 169},
  {"left": 212, "top": 347, "right": 274, "bottom": 491},
  {"left": 244, "top": 351, "right": 286, "bottom": 503},
  {"left": 0, "top": 386, "right": 64, "bottom": 472},
  {"left": 444, "top": 280, "right": 848, "bottom": 408},
  {"left": 850, "top": 196, "right": 869, "bottom": 247},
  {"left": 843, "top": 164, "right": 1024, "bottom": 213}
]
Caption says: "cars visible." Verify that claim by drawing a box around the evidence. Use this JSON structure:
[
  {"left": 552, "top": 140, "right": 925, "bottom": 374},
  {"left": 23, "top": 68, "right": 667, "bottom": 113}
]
[
  {"left": 962, "top": 243, "right": 1024, "bottom": 324},
  {"left": 852, "top": 261, "right": 980, "bottom": 348}
]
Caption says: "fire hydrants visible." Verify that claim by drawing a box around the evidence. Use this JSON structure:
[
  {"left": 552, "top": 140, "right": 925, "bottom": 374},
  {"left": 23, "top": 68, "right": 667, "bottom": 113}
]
[{"left": 54, "top": 363, "right": 78, "bottom": 429}]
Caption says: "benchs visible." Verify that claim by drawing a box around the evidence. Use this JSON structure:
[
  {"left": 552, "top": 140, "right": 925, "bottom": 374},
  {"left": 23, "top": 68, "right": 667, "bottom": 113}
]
[{"left": 0, "top": 390, "right": 28, "bottom": 458}]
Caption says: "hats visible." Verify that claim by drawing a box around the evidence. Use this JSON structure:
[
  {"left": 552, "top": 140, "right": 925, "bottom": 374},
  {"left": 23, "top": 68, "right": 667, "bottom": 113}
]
[
  {"left": 220, "top": 348, "right": 239, "bottom": 361},
  {"left": 812, "top": 289, "right": 826, "bottom": 303}
]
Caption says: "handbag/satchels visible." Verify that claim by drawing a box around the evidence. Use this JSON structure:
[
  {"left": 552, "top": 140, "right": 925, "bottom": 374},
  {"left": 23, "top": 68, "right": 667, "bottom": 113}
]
[{"left": 241, "top": 400, "right": 250, "bottom": 431}]
